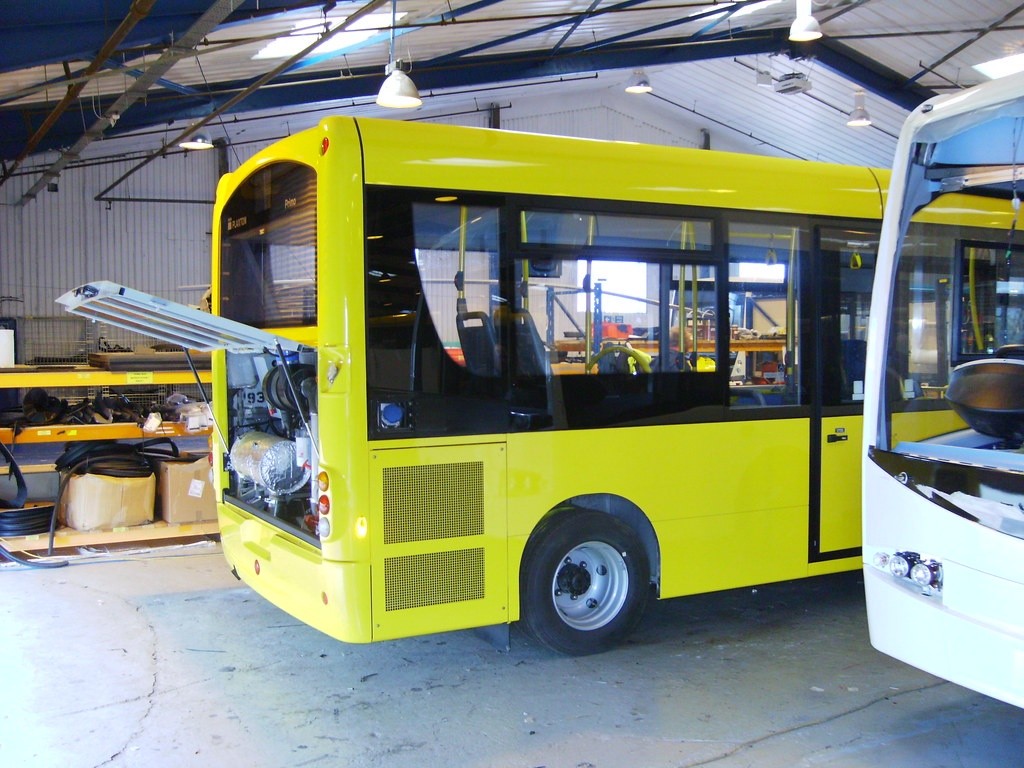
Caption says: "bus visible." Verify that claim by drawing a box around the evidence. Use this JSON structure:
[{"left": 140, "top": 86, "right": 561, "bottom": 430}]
[
  {"left": 54, "top": 115, "right": 1024, "bottom": 657},
  {"left": 862, "top": 70, "right": 1024, "bottom": 709}
]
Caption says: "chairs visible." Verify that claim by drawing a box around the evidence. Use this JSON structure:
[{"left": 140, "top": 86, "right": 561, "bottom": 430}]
[
  {"left": 586, "top": 345, "right": 691, "bottom": 375},
  {"left": 457, "top": 307, "right": 552, "bottom": 378},
  {"left": 840, "top": 340, "right": 867, "bottom": 392}
]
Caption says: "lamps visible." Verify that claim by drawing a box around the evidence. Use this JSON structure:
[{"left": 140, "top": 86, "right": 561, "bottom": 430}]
[
  {"left": 178, "top": 118, "right": 215, "bottom": 149},
  {"left": 625, "top": 70, "right": 652, "bottom": 93},
  {"left": 788, "top": 0, "right": 823, "bottom": 41},
  {"left": 847, "top": 93, "right": 871, "bottom": 127},
  {"left": 376, "top": 69, "right": 423, "bottom": 109}
]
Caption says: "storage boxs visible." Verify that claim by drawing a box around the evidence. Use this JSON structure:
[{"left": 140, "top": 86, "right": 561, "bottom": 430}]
[
  {"left": 60, "top": 471, "right": 157, "bottom": 531},
  {"left": 153, "top": 456, "right": 217, "bottom": 523}
]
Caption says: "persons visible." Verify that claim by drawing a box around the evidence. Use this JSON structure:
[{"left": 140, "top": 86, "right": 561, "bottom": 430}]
[{"left": 649, "top": 326, "right": 693, "bottom": 373}]
